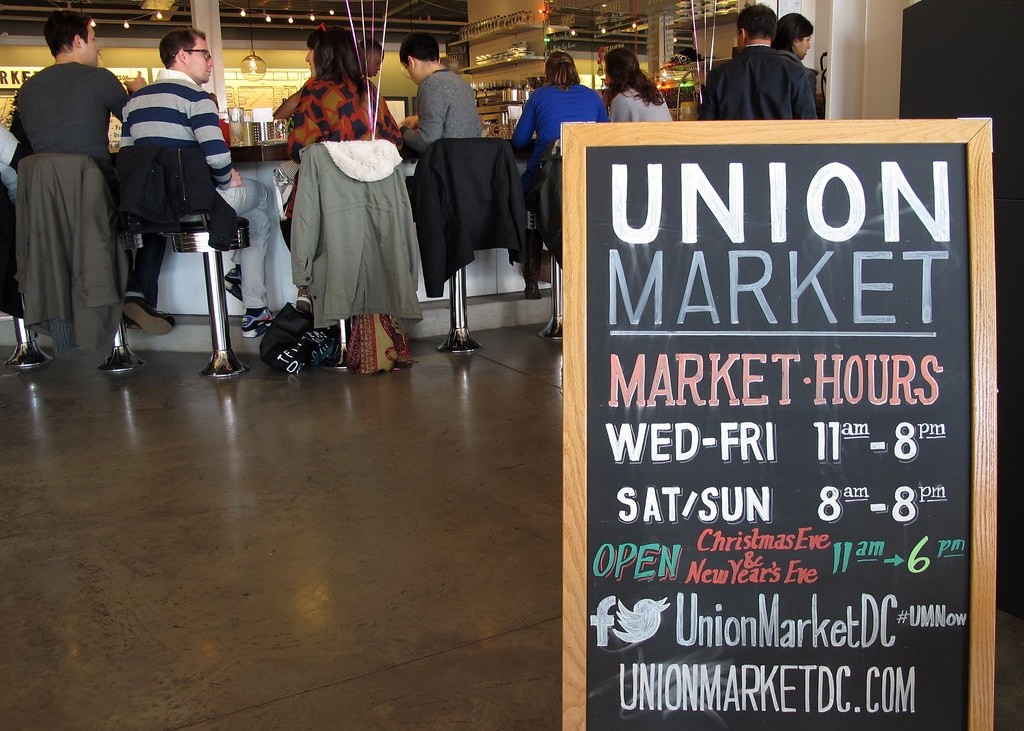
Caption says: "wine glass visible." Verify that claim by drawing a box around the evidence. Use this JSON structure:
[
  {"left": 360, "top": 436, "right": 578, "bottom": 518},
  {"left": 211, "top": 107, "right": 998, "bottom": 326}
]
[{"left": 226, "top": 106, "right": 245, "bottom": 146}]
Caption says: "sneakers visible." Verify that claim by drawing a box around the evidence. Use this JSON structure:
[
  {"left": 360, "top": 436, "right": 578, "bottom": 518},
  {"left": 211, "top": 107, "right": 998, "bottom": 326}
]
[
  {"left": 224, "top": 268, "right": 244, "bottom": 302},
  {"left": 241, "top": 308, "right": 275, "bottom": 338}
]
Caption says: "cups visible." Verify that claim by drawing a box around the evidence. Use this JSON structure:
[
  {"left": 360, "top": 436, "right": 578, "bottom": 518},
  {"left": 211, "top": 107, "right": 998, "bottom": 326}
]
[
  {"left": 125, "top": 77, "right": 144, "bottom": 95},
  {"left": 273, "top": 118, "right": 288, "bottom": 143},
  {"left": 486, "top": 124, "right": 510, "bottom": 138},
  {"left": 475, "top": 46, "right": 527, "bottom": 65},
  {"left": 469, "top": 79, "right": 515, "bottom": 93}
]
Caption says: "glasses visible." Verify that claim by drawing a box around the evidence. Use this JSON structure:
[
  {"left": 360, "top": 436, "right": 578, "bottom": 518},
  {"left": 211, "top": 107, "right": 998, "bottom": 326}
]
[
  {"left": 317, "top": 23, "right": 326, "bottom": 33},
  {"left": 173, "top": 49, "right": 211, "bottom": 63}
]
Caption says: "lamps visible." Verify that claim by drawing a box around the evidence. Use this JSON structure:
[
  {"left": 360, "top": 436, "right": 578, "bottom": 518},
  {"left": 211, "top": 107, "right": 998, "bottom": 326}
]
[{"left": 241, "top": 1, "right": 266, "bottom": 81}]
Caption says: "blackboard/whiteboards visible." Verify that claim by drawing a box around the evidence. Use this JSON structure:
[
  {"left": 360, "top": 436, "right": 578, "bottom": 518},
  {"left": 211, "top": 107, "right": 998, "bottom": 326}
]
[{"left": 559, "top": 117, "right": 998, "bottom": 731}]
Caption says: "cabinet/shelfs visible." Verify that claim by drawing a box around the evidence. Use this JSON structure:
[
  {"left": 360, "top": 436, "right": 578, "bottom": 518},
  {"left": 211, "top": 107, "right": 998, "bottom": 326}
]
[
  {"left": 667, "top": 10, "right": 738, "bottom": 71},
  {"left": 449, "top": 24, "right": 546, "bottom": 136}
]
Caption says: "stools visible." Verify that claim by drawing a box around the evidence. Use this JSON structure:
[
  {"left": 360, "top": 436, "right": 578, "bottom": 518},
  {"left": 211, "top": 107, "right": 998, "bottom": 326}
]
[{"left": 0, "top": 215, "right": 563, "bottom": 381}]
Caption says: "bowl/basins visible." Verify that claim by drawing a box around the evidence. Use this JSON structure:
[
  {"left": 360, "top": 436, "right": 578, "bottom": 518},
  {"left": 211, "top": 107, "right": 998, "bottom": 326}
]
[{"left": 526, "top": 77, "right": 544, "bottom": 89}]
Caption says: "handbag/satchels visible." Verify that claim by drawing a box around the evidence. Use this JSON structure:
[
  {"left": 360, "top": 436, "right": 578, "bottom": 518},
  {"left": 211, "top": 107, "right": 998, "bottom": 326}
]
[{"left": 258, "top": 301, "right": 341, "bottom": 374}]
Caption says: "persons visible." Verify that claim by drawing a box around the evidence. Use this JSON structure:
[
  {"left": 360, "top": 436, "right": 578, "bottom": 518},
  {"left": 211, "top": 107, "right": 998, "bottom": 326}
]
[
  {"left": 604, "top": 48, "right": 675, "bottom": 121},
  {"left": 285, "top": 26, "right": 403, "bottom": 218},
  {"left": 771, "top": 13, "right": 814, "bottom": 60},
  {"left": 397, "top": 31, "right": 483, "bottom": 152},
  {"left": 511, "top": 51, "right": 609, "bottom": 299},
  {"left": 698, "top": 5, "right": 818, "bottom": 120},
  {"left": 16, "top": 10, "right": 175, "bottom": 335},
  {"left": 121, "top": 30, "right": 277, "bottom": 337},
  {"left": 272, "top": 38, "right": 384, "bottom": 120}
]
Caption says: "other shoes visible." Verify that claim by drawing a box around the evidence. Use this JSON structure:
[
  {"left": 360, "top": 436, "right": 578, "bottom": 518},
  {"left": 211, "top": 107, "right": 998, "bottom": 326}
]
[
  {"left": 122, "top": 296, "right": 175, "bottom": 336},
  {"left": 524, "top": 281, "right": 542, "bottom": 300}
]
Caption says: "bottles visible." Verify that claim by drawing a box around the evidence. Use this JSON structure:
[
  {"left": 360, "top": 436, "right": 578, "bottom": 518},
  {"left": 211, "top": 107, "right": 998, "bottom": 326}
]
[
  {"left": 502, "top": 84, "right": 530, "bottom": 101},
  {"left": 460, "top": 10, "right": 534, "bottom": 41},
  {"left": 244, "top": 113, "right": 255, "bottom": 146}
]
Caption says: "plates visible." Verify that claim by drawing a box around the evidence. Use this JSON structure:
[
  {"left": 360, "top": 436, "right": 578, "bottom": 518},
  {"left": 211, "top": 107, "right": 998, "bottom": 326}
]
[{"left": 676, "top": 0, "right": 738, "bottom": 22}]
